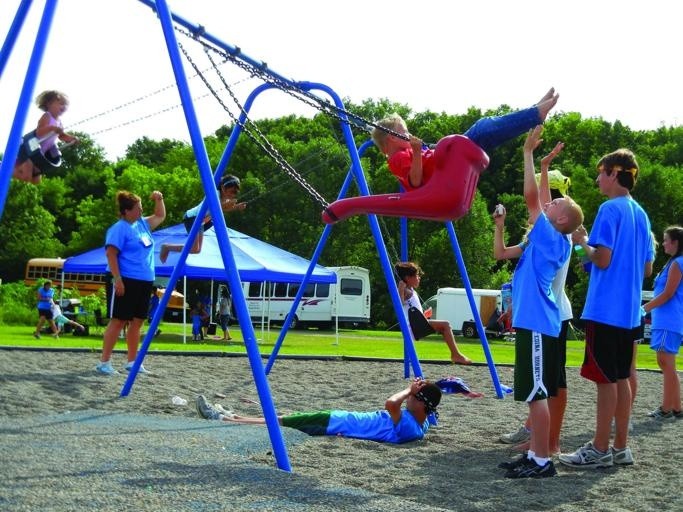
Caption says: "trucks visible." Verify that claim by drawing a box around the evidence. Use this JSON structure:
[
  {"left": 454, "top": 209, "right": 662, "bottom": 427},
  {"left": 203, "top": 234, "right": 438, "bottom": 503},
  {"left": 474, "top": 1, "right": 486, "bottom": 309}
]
[
  {"left": 241, "top": 266, "right": 371, "bottom": 329},
  {"left": 641, "top": 290, "right": 658, "bottom": 339},
  {"left": 422, "top": 287, "right": 503, "bottom": 338}
]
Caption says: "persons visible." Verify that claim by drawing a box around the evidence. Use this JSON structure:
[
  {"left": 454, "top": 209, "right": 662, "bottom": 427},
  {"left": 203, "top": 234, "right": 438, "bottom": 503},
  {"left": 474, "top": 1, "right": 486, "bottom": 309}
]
[
  {"left": 8, "top": 90, "right": 81, "bottom": 185},
  {"left": 395, "top": 261, "right": 474, "bottom": 365},
  {"left": 644, "top": 226, "right": 683, "bottom": 422},
  {"left": 195, "top": 378, "right": 442, "bottom": 444},
  {"left": 559, "top": 149, "right": 653, "bottom": 469},
  {"left": 497, "top": 123, "right": 585, "bottom": 479},
  {"left": 218, "top": 286, "right": 232, "bottom": 340},
  {"left": 371, "top": 86, "right": 560, "bottom": 192},
  {"left": 612, "top": 229, "right": 660, "bottom": 431},
  {"left": 96, "top": 189, "right": 169, "bottom": 374},
  {"left": 493, "top": 169, "right": 573, "bottom": 455},
  {"left": 149, "top": 288, "right": 162, "bottom": 337},
  {"left": 50, "top": 299, "right": 85, "bottom": 340},
  {"left": 160, "top": 175, "right": 250, "bottom": 263},
  {"left": 190, "top": 292, "right": 211, "bottom": 341},
  {"left": 33, "top": 282, "right": 59, "bottom": 340},
  {"left": 502, "top": 298, "right": 512, "bottom": 332}
]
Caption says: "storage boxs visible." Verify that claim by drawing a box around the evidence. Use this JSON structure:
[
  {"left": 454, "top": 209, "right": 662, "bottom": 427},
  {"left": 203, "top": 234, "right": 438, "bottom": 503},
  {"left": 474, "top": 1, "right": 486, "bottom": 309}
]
[{"left": 55, "top": 299, "right": 81, "bottom": 313}]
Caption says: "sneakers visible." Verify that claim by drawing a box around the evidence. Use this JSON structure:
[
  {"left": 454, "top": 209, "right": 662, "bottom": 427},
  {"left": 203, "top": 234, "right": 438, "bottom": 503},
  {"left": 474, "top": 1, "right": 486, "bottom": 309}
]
[
  {"left": 499, "top": 424, "right": 530, "bottom": 444},
  {"left": 647, "top": 407, "right": 673, "bottom": 421},
  {"left": 611, "top": 446, "right": 633, "bottom": 465},
  {"left": 214, "top": 403, "right": 232, "bottom": 417},
  {"left": 126, "top": 362, "right": 144, "bottom": 373},
  {"left": 97, "top": 366, "right": 115, "bottom": 374},
  {"left": 558, "top": 441, "right": 613, "bottom": 469},
  {"left": 672, "top": 408, "right": 683, "bottom": 417},
  {"left": 505, "top": 458, "right": 556, "bottom": 479},
  {"left": 498, "top": 454, "right": 529, "bottom": 468},
  {"left": 196, "top": 395, "right": 214, "bottom": 419}
]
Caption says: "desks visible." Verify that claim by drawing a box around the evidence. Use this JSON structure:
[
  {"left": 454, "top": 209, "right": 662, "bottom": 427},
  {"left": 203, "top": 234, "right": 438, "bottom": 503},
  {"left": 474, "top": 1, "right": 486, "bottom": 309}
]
[{"left": 61, "top": 311, "right": 89, "bottom": 335}]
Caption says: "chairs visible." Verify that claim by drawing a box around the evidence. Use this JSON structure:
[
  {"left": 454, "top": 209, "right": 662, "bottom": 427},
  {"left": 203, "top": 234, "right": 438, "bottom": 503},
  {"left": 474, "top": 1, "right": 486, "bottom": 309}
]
[{"left": 95, "top": 309, "right": 113, "bottom": 336}]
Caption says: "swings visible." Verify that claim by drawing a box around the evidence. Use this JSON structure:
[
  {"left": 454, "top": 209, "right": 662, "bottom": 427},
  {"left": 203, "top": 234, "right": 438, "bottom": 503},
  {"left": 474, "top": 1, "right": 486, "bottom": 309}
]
[
  {"left": 18, "top": 52, "right": 258, "bottom": 183},
  {"left": 173, "top": 25, "right": 490, "bottom": 225},
  {"left": 182, "top": 129, "right": 366, "bottom": 235},
  {"left": 378, "top": 216, "right": 435, "bottom": 342}
]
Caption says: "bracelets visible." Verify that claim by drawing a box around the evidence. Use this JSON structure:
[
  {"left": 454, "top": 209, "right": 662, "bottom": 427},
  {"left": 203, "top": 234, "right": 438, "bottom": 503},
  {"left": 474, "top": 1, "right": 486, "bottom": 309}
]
[
  {"left": 159, "top": 195, "right": 165, "bottom": 201},
  {"left": 110, "top": 275, "right": 121, "bottom": 284},
  {"left": 639, "top": 306, "right": 647, "bottom": 319}
]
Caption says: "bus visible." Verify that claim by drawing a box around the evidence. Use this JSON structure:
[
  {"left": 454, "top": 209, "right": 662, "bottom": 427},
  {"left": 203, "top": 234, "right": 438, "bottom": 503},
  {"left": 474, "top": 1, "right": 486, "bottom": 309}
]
[{"left": 25, "top": 257, "right": 191, "bottom": 322}]
